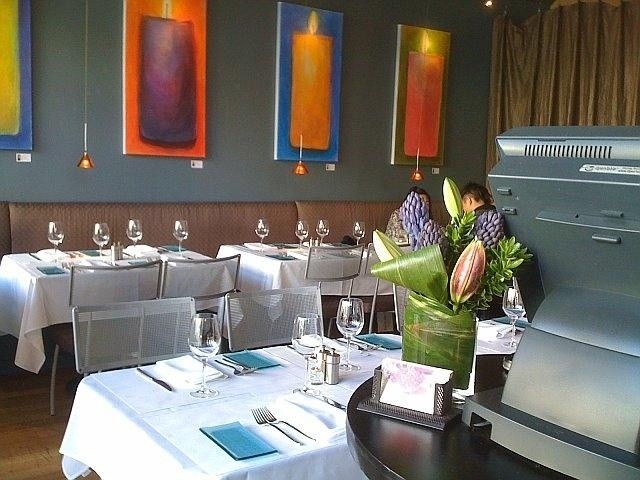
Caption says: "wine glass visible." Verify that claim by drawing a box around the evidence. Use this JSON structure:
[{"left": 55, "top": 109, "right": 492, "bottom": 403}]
[
  {"left": 244, "top": 219, "right": 373, "bottom": 259},
  {"left": 502, "top": 286, "right": 525, "bottom": 349},
  {"left": 44, "top": 218, "right": 190, "bottom": 270},
  {"left": 185, "top": 298, "right": 374, "bottom": 399}
]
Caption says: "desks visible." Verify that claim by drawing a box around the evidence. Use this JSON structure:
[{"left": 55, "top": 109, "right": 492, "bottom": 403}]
[{"left": 347, "top": 353, "right": 640, "bottom": 480}]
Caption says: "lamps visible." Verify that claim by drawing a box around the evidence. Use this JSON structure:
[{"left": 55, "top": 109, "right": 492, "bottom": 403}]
[
  {"left": 77, "top": 0, "right": 95, "bottom": 169},
  {"left": 294, "top": 134, "right": 310, "bottom": 175},
  {"left": 411, "top": 0, "right": 430, "bottom": 181}
]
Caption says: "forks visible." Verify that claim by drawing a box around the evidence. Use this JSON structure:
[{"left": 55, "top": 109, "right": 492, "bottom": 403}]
[{"left": 251, "top": 406, "right": 317, "bottom": 446}]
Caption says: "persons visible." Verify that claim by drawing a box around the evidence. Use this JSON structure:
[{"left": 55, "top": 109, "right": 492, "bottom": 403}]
[
  {"left": 384, "top": 186, "right": 434, "bottom": 245},
  {"left": 460, "top": 182, "right": 496, "bottom": 219}
]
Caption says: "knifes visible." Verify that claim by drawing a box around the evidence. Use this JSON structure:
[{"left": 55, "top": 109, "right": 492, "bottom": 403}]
[{"left": 136, "top": 365, "right": 172, "bottom": 394}]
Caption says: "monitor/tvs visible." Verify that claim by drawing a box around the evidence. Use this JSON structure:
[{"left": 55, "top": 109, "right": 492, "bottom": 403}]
[{"left": 461, "top": 126, "right": 640, "bottom": 480}]
